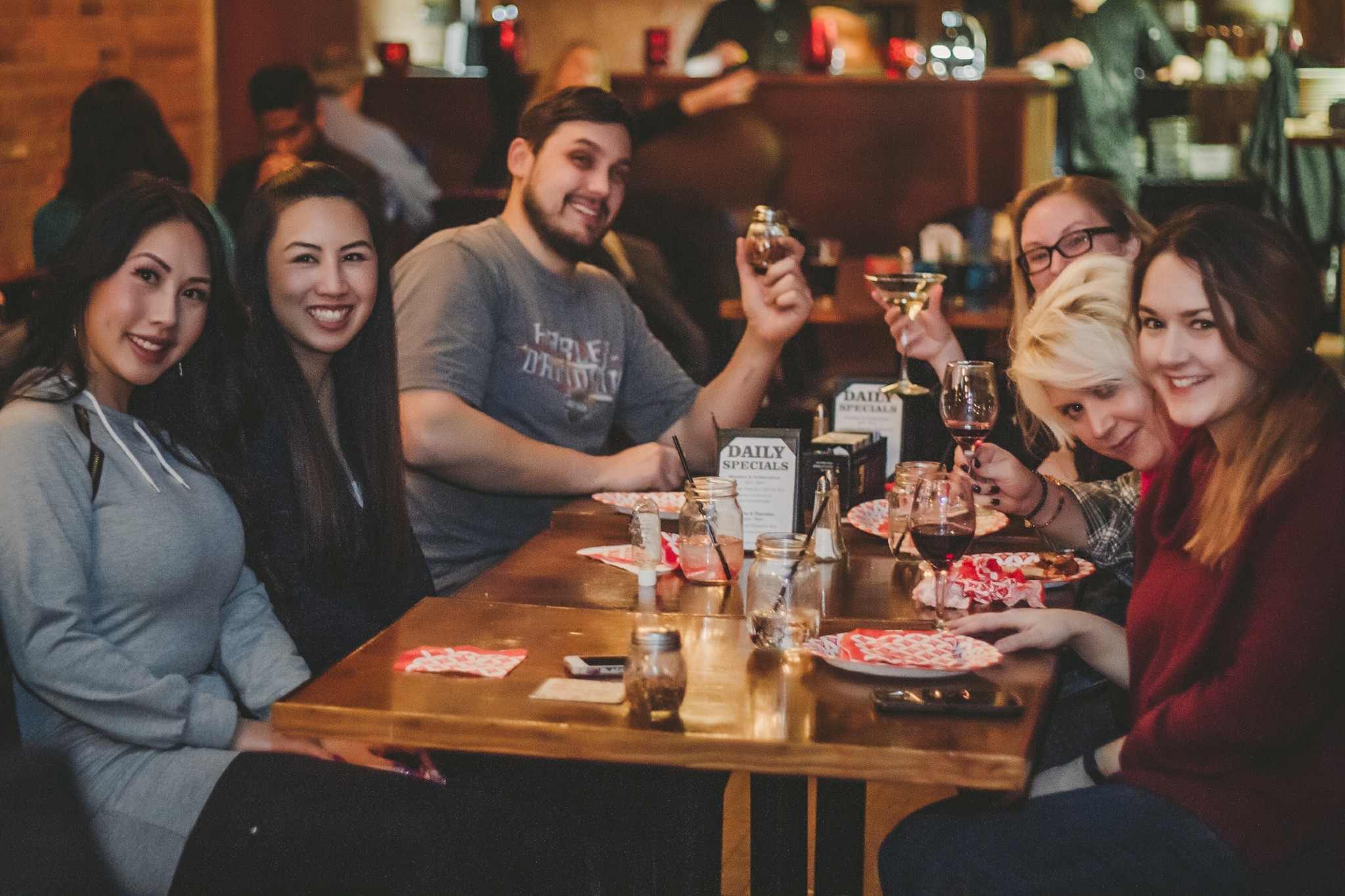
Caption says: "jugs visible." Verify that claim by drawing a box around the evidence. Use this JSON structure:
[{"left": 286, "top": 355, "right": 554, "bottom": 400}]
[{"left": 927, "top": 12, "right": 986, "bottom": 80}]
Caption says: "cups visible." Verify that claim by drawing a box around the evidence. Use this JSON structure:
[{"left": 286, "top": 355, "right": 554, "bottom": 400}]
[
  {"left": 643, "top": 27, "right": 670, "bottom": 76},
  {"left": 801, "top": 238, "right": 840, "bottom": 299}
]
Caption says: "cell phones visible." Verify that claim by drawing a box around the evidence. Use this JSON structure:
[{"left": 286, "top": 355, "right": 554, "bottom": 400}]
[
  {"left": 872, "top": 689, "right": 1024, "bottom": 715},
  {"left": 564, "top": 656, "right": 629, "bottom": 676}
]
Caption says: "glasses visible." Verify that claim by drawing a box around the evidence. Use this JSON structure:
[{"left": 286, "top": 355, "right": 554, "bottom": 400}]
[{"left": 1015, "top": 226, "right": 1120, "bottom": 275}]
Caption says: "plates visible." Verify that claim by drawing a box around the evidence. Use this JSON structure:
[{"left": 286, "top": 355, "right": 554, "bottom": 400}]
[
  {"left": 590, "top": 491, "right": 686, "bottom": 520},
  {"left": 847, "top": 497, "right": 1009, "bottom": 540},
  {"left": 800, "top": 629, "right": 1007, "bottom": 676},
  {"left": 917, "top": 550, "right": 1103, "bottom": 583}
]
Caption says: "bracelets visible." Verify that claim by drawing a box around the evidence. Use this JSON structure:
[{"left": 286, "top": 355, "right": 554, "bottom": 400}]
[
  {"left": 1083, "top": 749, "right": 1107, "bottom": 784},
  {"left": 1020, "top": 468, "right": 1048, "bottom": 521},
  {"left": 1030, "top": 473, "right": 1065, "bottom": 528}
]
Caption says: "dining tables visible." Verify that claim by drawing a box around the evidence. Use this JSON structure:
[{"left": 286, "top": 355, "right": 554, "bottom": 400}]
[{"left": 269, "top": 294, "right": 1345, "bottom": 896}]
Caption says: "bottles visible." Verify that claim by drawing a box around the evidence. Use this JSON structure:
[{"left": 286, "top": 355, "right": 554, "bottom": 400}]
[
  {"left": 742, "top": 206, "right": 790, "bottom": 270},
  {"left": 622, "top": 625, "right": 687, "bottom": 727},
  {"left": 887, "top": 461, "right": 948, "bottom": 567},
  {"left": 810, "top": 403, "right": 831, "bottom": 439},
  {"left": 811, "top": 469, "right": 849, "bottom": 563},
  {"left": 677, "top": 476, "right": 745, "bottom": 585},
  {"left": 745, "top": 532, "right": 823, "bottom": 654},
  {"left": 631, "top": 497, "right": 662, "bottom": 587}
]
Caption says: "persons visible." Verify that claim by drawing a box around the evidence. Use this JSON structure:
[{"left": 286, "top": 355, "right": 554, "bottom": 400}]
[
  {"left": 684, "top": 0, "right": 814, "bottom": 77},
  {"left": 873, "top": 176, "right": 1344, "bottom": 896},
  {"left": 1017, "top": 1, "right": 1201, "bottom": 212},
  {"left": 1, "top": 41, "right": 813, "bottom": 895}
]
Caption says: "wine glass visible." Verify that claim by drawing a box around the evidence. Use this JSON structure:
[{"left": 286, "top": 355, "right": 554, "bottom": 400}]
[
  {"left": 908, "top": 472, "right": 977, "bottom": 632},
  {"left": 864, "top": 272, "right": 946, "bottom": 395},
  {"left": 939, "top": 360, "right": 1000, "bottom": 478}
]
